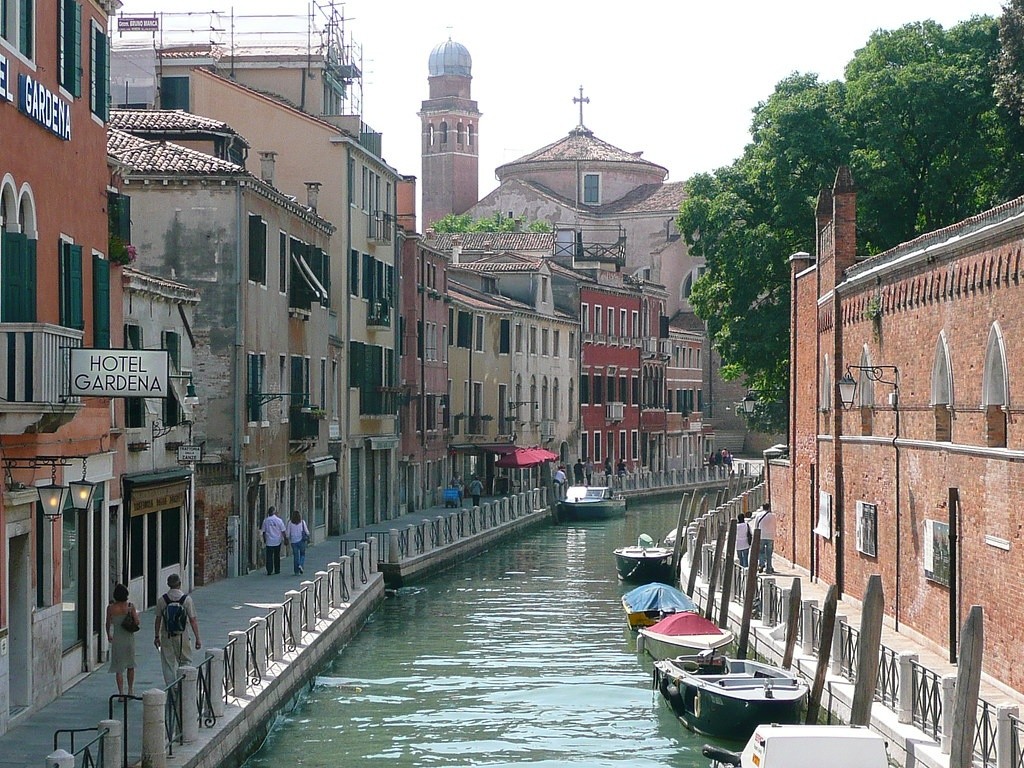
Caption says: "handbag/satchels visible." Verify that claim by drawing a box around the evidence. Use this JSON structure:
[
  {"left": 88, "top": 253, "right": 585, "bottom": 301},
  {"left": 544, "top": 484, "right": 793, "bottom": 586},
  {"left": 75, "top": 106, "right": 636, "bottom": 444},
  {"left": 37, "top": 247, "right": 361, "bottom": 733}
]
[
  {"left": 120, "top": 602, "right": 140, "bottom": 632},
  {"left": 301, "top": 520, "right": 309, "bottom": 541},
  {"left": 747, "top": 524, "right": 752, "bottom": 545}
]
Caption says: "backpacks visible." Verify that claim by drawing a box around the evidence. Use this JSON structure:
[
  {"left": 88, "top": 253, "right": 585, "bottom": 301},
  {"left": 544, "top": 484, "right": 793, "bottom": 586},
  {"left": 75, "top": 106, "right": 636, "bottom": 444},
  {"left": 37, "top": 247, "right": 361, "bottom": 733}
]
[{"left": 161, "top": 593, "right": 188, "bottom": 635}]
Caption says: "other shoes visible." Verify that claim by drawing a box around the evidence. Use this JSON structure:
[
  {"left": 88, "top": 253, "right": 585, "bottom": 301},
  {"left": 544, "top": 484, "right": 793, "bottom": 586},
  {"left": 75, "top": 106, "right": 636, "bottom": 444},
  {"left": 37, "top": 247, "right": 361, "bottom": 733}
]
[
  {"left": 295, "top": 573, "right": 299, "bottom": 575},
  {"left": 759, "top": 565, "right": 765, "bottom": 573},
  {"left": 768, "top": 568, "right": 773, "bottom": 571},
  {"left": 298, "top": 565, "right": 303, "bottom": 574}
]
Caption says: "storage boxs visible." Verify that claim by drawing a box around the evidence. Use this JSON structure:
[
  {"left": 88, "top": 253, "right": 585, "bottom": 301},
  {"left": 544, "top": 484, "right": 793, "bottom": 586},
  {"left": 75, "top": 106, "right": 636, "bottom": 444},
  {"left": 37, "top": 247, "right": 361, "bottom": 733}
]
[{"left": 443, "top": 487, "right": 459, "bottom": 504}]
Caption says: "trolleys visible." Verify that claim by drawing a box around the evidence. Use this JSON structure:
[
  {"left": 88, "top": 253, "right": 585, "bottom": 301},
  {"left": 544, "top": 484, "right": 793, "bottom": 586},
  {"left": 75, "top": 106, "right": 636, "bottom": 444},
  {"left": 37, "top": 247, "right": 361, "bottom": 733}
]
[{"left": 445, "top": 484, "right": 461, "bottom": 508}]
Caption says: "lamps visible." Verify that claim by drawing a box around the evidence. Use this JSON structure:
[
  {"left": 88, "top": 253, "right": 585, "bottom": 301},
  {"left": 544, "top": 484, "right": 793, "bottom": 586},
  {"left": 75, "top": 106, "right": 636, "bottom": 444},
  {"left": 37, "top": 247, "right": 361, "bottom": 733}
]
[
  {"left": 1, "top": 457, "right": 72, "bottom": 521},
  {"left": 42, "top": 456, "right": 98, "bottom": 510},
  {"left": 393, "top": 392, "right": 445, "bottom": 409},
  {"left": 508, "top": 401, "right": 539, "bottom": 411},
  {"left": 837, "top": 364, "right": 897, "bottom": 412},
  {"left": 743, "top": 387, "right": 789, "bottom": 414},
  {"left": 169, "top": 373, "right": 199, "bottom": 405},
  {"left": 247, "top": 392, "right": 312, "bottom": 421}
]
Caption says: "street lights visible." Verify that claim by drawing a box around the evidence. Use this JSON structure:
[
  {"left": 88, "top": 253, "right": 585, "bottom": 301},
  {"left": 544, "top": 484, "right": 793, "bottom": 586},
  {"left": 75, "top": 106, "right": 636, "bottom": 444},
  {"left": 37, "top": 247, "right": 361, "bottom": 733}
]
[
  {"left": 837, "top": 363, "right": 899, "bottom": 631},
  {"left": 743, "top": 389, "right": 789, "bottom": 457}
]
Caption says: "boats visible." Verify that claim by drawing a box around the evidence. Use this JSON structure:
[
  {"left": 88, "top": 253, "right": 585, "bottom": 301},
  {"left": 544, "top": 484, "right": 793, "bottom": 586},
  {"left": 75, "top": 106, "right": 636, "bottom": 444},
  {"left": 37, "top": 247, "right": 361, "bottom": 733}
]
[
  {"left": 614, "top": 538, "right": 888, "bottom": 768},
  {"left": 556, "top": 486, "right": 627, "bottom": 522}
]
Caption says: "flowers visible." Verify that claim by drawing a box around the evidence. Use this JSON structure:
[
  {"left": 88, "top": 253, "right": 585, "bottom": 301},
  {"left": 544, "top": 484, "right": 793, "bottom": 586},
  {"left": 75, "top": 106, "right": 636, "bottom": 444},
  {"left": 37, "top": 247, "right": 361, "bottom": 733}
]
[{"left": 109, "top": 234, "right": 138, "bottom": 267}]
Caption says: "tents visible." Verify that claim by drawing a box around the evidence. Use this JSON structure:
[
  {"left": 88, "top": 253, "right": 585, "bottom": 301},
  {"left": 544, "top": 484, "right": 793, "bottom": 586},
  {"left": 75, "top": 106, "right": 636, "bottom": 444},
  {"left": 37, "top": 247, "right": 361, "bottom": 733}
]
[{"left": 495, "top": 444, "right": 559, "bottom": 492}]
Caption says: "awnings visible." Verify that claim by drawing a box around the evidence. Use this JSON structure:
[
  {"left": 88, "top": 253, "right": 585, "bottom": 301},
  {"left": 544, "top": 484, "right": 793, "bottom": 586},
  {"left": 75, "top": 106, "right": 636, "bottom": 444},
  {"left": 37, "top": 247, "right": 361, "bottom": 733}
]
[{"left": 474, "top": 443, "right": 519, "bottom": 455}]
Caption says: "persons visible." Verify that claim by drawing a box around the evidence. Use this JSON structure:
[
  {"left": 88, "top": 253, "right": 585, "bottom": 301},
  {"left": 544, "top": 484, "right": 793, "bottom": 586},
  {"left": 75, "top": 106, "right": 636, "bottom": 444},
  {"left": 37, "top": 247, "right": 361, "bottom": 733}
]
[
  {"left": 105, "top": 583, "right": 141, "bottom": 701},
  {"left": 573, "top": 458, "right": 585, "bottom": 486},
  {"left": 468, "top": 475, "right": 484, "bottom": 507},
  {"left": 552, "top": 463, "right": 568, "bottom": 498},
  {"left": 286, "top": 510, "right": 311, "bottom": 575},
  {"left": 583, "top": 457, "right": 594, "bottom": 486},
  {"left": 261, "top": 506, "right": 288, "bottom": 576},
  {"left": 707, "top": 446, "right": 734, "bottom": 474},
  {"left": 449, "top": 472, "right": 465, "bottom": 507},
  {"left": 603, "top": 456, "right": 615, "bottom": 486},
  {"left": 617, "top": 458, "right": 631, "bottom": 489},
  {"left": 735, "top": 513, "right": 753, "bottom": 568},
  {"left": 153, "top": 574, "right": 201, "bottom": 703},
  {"left": 755, "top": 502, "right": 778, "bottom": 574}
]
